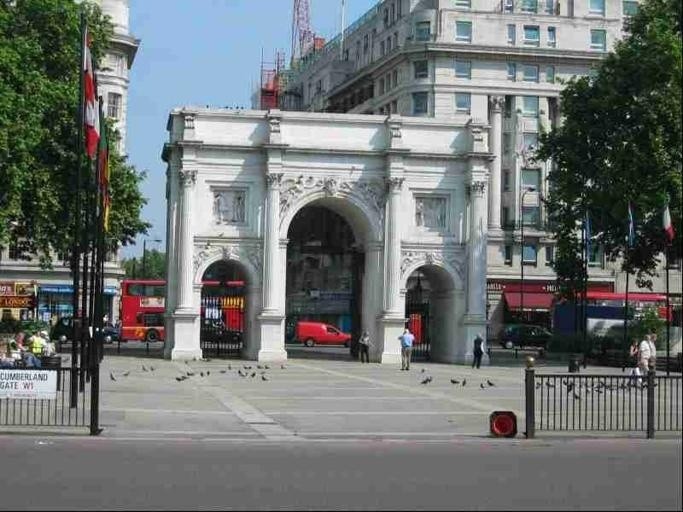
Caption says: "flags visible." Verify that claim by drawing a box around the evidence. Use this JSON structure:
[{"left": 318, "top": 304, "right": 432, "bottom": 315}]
[
  {"left": 660, "top": 202, "right": 677, "bottom": 245},
  {"left": 625, "top": 196, "right": 637, "bottom": 251},
  {"left": 78, "top": 25, "right": 115, "bottom": 238},
  {"left": 581, "top": 207, "right": 592, "bottom": 262}
]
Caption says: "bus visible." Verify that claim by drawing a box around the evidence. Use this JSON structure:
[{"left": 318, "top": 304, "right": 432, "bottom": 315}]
[
  {"left": 549, "top": 289, "right": 682, "bottom": 356},
  {"left": 113, "top": 278, "right": 250, "bottom": 343},
  {"left": 0, "top": 279, "right": 39, "bottom": 353}
]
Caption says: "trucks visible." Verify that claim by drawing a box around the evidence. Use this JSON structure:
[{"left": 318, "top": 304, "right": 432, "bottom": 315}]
[{"left": 292, "top": 321, "right": 352, "bottom": 347}]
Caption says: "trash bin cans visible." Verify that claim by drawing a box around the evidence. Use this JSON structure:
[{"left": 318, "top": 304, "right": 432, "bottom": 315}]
[{"left": 41, "top": 356, "right": 62, "bottom": 392}]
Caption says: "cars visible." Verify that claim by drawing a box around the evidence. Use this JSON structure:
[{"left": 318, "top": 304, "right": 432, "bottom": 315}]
[
  {"left": 47, "top": 316, "right": 129, "bottom": 347},
  {"left": 497, "top": 322, "right": 555, "bottom": 349},
  {"left": 199, "top": 317, "right": 243, "bottom": 343}
]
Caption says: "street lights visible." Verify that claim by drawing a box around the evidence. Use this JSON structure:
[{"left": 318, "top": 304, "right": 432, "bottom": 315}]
[
  {"left": 140, "top": 238, "right": 162, "bottom": 296},
  {"left": 518, "top": 184, "right": 536, "bottom": 322}
]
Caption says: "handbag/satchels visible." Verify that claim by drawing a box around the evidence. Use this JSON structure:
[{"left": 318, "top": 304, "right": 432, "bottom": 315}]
[{"left": 624, "top": 356, "right": 638, "bottom": 367}]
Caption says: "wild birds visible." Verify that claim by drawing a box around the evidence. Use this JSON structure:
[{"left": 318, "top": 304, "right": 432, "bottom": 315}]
[
  {"left": 573, "top": 394, "right": 580, "bottom": 400},
  {"left": 487, "top": 380, "right": 494, "bottom": 386},
  {"left": 480, "top": 383, "right": 484, "bottom": 388},
  {"left": 421, "top": 376, "right": 434, "bottom": 384},
  {"left": 109, "top": 355, "right": 272, "bottom": 383},
  {"left": 535, "top": 380, "right": 619, "bottom": 393},
  {"left": 449, "top": 378, "right": 459, "bottom": 384},
  {"left": 462, "top": 379, "right": 466, "bottom": 386}
]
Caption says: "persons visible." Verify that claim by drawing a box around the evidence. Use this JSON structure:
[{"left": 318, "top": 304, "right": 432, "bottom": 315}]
[
  {"left": 470, "top": 332, "right": 485, "bottom": 370},
  {"left": 397, "top": 328, "right": 416, "bottom": 370},
  {"left": 650, "top": 334, "right": 657, "bottom": 387},
  {"left": 638, "top": 333, "right": 657, "bottom": 388},
  {"left": 0, "top": 307, "right": 123, "bottom": 369},
  {"left": 357, "top": 331, "right": 374, "bottom": 364},
  {"left": 619, "top": 338, "right": 644, "bottom": 390}
]
